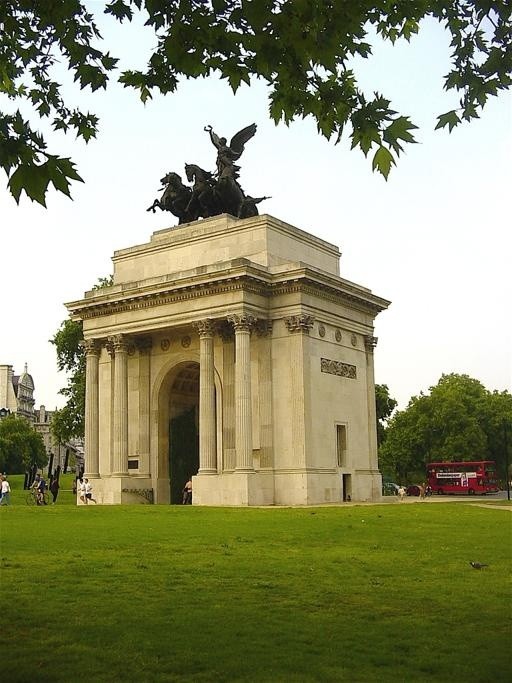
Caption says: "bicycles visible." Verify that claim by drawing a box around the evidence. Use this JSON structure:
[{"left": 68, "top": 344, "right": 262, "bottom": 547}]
[{"left": 26, "top": 487, "right": 50, "bottom": 505}]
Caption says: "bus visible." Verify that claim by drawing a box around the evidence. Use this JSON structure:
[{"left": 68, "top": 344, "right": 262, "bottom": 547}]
[{"left": 424, "top": 461, "right": 498, "bottom": 496}]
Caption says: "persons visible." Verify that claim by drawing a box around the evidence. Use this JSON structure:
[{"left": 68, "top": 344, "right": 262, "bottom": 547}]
[
  {"left": 77, "top": 478, "right": 86, "bottom": 504},
  {"left": 72, "top": 479, "right": 77, "bottom": 494},
  {"left": 397, "top": 481, "right": 432, "bottom": 501},
  {"left": 51, "top": 476, "right": 61, "bottom": 505},
  {"left": 181, "top": 484, "right": 190, "bottom": 504},
  {"left": 37, "top": 475, "right": 49, "bottom": 506},
  {"left": 209, "top": 129, "right": 243, "bottom": 180},
  {"left": 184, "top": 477, "right": 192, "bottom": 505},
  {"left": 29, "top": 472, "right": 45, "bottom": 504},
  {"left": 0, "top": 470, "right": 13, "bottom": 507},
  {"left": 82, "top": 478, "right": 98, "bottom": 505}
]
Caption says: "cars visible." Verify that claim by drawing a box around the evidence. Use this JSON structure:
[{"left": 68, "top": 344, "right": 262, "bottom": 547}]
[{"left": 385, "top": 483, "right": 419, "bottom": 496}]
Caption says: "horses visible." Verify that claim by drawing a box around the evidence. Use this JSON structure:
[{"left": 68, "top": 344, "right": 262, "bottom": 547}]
[
  {"left": 184, "top": 163, "right": 226, "bottom": 218},
  {"left": 146, "top": 172, "right": 198, "bottom": 225},
  {"left": 210, "top": 157, "right": 258, "bottom": 218}
]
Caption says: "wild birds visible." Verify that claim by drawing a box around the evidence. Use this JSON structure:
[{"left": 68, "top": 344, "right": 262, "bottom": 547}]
[{"left": 469, "top": 562, "right": 488, "bottom": 569}]
